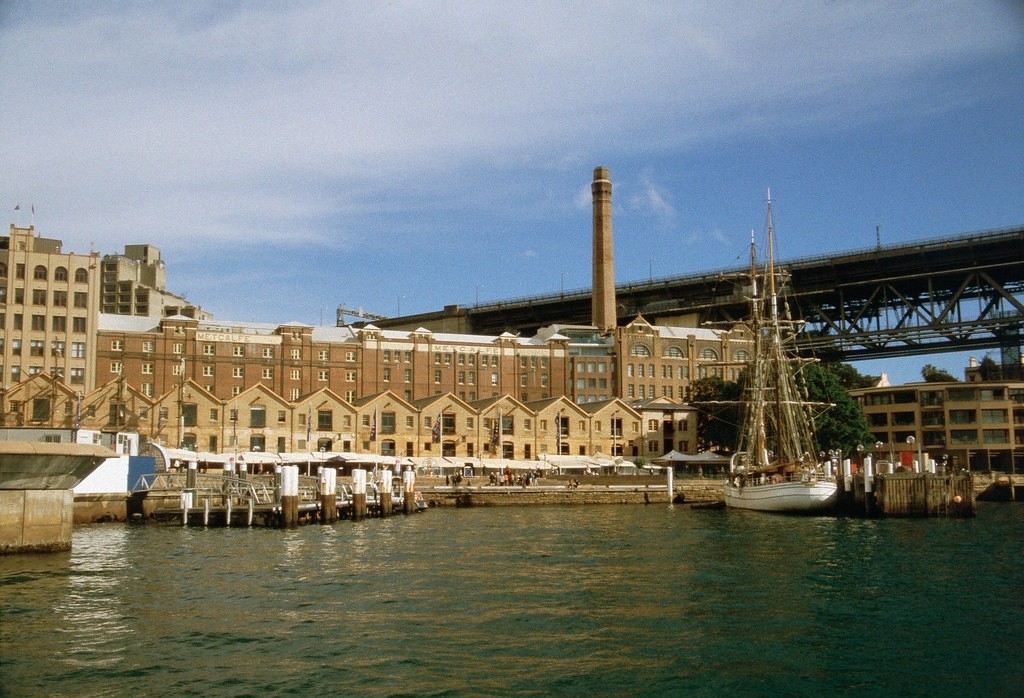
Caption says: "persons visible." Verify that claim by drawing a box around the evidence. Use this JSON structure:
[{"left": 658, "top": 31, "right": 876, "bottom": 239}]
[
  {"left": 586, "top": 465, "right": 591, "bottom": 475},
  {"left": 721, "top": 467, "right": 725, "bottom": 480},
  {"left": 174, "top": 458, "right": 207, "bottom": 474},
  {"left": 649, "top": 467, "right": 654, "bottom": 475},
  {"left": 698, "top": 466, "right": 705, "bottom": 479},
  {"left": 734, "top": 474, "right": 741, "bottom": 487},
  {"left": 446, "top": 464, "right": 578, "bottom": 492},
  {"left": 166, "top": 467, "right": 173, "bottom": 488},
  {"left": 946, "top": 454, "right": 953, "bottom": 485},
  {"left": 271, "top": 461, "right": 277, "bottom": 474}
]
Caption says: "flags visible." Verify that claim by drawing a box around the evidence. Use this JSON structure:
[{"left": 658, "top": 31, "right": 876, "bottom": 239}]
[
  {"left": 491, "top": 418, "right": 499, "bottom": 446},
  {"left": 14, "top": 205, "right": 20, "bottom": 210},
  {"left": 370, "top": 410, "right": 376, "bottom": 441},
  {"left": 307, "top": 406, "right": 311, "bottom": 442},
  {"left": 31, "top": 205, "right": 35, "bottom": 214},
  {"left": 432, "top": 415, "right": 440, "bottom": 443}
]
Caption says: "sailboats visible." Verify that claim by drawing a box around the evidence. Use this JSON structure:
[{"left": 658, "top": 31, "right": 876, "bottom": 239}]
[{"left": 690, "top": 183, "right": 841, "bottom": 512}]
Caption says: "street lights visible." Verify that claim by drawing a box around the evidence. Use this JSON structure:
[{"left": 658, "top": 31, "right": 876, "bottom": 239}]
[
  {"left": 943, "top": 454, "right": 948, "bottom": 473},
  {"left": 836, "top": 449, "right": 842, "bottom": 477},
  {"left": 320, "top": 308, "right": 329, "bottom": 326},
  {"left": 857, "top": 444, "right": 865, "bottom": 474},
  {"left": 558, "top": 408, "right": 565, "bottom": 475},
  {"left": 392, "top": 296, "right": 406, "bottom": 317},
  {"left": 613, "top": 410, "right": 620, "bottom": 473},
  {"left": 907, "top": 435, "right": 916, "bottom": 472},
  {"left": 819, "top": 451, "right": 826, "bottom": 476},
  {"left": 649, "top": 259, "right": 656, "bottom": 283},
  {"left": 669, "top": 453, "right": 673, "bottom": 467},
  {"left": 471, "top": 285, "right": 484, "bottom": 305},
  {"left": 875, "top": 441, "right": 883, "bottom": 473},
  {"left": 828, "top": 449, "right": 835, "bottom": 477},
  {"left": 561, "top": 273, "right": 568, "bottom": 294}
]
